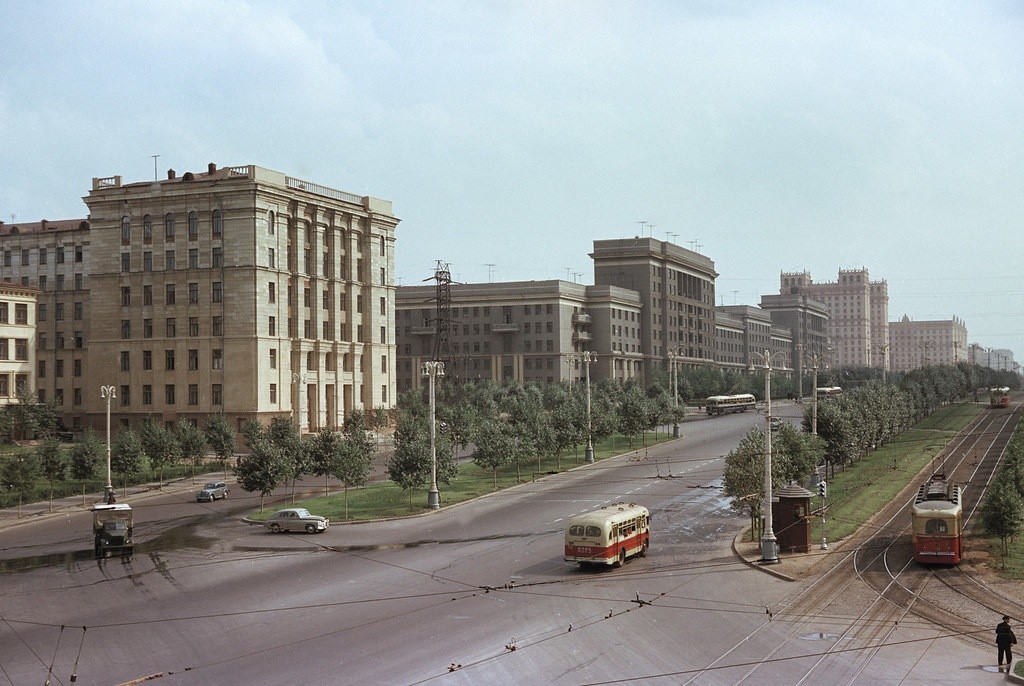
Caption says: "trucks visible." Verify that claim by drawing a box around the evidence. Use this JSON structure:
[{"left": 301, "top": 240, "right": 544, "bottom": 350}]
[{"left": 93, "top": 503, "right": 134, "bottom": 557}]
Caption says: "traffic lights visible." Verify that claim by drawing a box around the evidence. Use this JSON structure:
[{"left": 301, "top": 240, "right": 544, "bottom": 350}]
[
  {"left": 817, "top": 481, "right": 827, "bottom": 498},
  {"left": 440, "top": 423, "right": 448, "bottom": 435}
]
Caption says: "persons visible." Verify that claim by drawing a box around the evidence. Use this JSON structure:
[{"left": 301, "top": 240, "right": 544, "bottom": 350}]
[
  {"left": 290, "top": 513, "right": 296, "bottom": 518},
  {"left": 108, "top": 492, "right": 116, "bottom": 505},
  {"left": 996, "top": 615, "right": 1017, "bottom": 674}
]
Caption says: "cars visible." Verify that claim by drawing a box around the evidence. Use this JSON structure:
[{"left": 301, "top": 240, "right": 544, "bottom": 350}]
[
  {"left": 196, "top": 481, "right": 230, "bottom": 500},
  {"left": 264, "top": 508, "right": 329, "bottom": 534}
]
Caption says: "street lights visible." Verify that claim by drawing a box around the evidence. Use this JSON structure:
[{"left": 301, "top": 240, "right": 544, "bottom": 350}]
[
  {"left": 749, "top": 352, "right": 786, "bottom": 565},
  {"left": 871, "top": 342, "right": 895, "bottom": 384},
  {"left": 801, "top": 354, "right": 830, "bottom": 486},
  {"left": 293, "top": 372, "right": 308, "bottom": 443},
  {"left": 419, "top": 359, "right": 446, "bottom": 510},
  {"left": 667, "top": 344, "right": 686, "bottom": 437},
  {"left": 566, "top": 351, "right": 598, "bottom": 462},
  {"left": 100, "top": 384, "right": 117, "bottom": 502},
  {"left": 919, "top": 339, "right": 1022, "bottom": 376}
]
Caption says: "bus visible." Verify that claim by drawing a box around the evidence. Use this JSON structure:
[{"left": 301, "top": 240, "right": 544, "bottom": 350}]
[
  {"left": 704, "top": 394, "right": 756, "bottom": 415},
  {"left": 912, "top": 478, "right": 964, "bottom": 564},
  {"left": 563, "top": 502, "right": 650, "bottom": 569},
  {"left": 817, "top": 386, "right": 842, "bottom": 400},
  {"left": 990, "top": 386, "right": 1011, "bottom": 408}
]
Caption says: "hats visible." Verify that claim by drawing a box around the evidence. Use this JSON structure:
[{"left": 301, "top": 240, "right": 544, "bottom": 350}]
[{"left": 1002, "top": 615, "right": 1009, "bottom": 620}]
